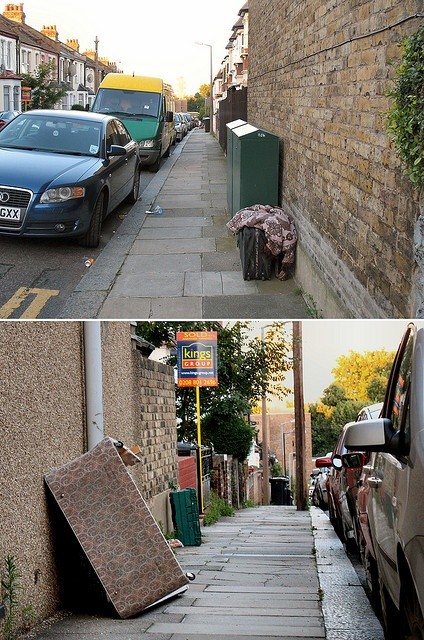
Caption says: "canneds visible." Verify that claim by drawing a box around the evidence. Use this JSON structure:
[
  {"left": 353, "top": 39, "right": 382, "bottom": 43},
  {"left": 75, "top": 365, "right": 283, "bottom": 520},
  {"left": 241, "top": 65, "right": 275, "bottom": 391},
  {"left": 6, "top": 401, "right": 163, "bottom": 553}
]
[{"left": 84, "top": 258, "right": 94, "bottom": 268}]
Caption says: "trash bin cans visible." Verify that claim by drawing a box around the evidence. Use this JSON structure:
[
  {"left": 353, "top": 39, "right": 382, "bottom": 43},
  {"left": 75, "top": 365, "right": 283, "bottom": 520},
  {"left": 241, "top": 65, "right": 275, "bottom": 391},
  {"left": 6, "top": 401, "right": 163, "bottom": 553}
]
[
  {"left": 225, "top": 119, "right": 248, "bottom": 219},
  {"left": 231, "top": 123, "right": 279, "bottom": 217},
  {"left": 205, "top": 118, "right": 210, "bottom": 132},
  {"left": 269, "top": 476, "right": 293, "bottom": 505}
]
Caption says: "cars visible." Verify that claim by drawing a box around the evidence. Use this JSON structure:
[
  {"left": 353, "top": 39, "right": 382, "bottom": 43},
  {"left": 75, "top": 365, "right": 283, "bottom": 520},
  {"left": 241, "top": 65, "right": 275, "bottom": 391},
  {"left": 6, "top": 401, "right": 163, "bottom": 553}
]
[
  {"left": 311, "top": 401, "right": 384, "bottom": 551},
  {"left": 342, "top": 321, "right": 424, "bottom": 639},
  {"left": 0, "top": 111, "right": 22, "bottom": 132},
  {"left": 175, "top": 112, "right": 200, "bottom": 141},
  {"left": 0, "top": 109, "right": 142, "bottom": 247}
]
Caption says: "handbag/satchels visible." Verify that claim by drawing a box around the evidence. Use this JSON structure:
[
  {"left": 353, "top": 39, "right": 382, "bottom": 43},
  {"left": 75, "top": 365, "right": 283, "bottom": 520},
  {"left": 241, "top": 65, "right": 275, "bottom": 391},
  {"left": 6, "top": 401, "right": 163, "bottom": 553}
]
[{"left": 235, "top": 224, "right": 270, "bottom": 281}]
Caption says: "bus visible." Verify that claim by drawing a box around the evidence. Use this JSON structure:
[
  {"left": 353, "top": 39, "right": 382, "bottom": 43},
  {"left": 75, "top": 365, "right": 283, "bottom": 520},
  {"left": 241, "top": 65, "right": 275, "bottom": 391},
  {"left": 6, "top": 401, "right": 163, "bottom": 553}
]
[{"left": 91, "top": 73, "right": 176, "bottom": 172}]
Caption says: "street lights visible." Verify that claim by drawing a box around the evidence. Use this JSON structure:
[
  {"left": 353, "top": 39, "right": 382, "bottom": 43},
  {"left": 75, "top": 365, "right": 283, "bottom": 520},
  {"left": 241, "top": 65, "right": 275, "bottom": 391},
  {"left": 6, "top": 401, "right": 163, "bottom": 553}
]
[
  {"left": 196, "top": 41, "right": 214, "bottom": 134},
  {"left": 256, "top": 319, "right": 279, "bottom": 514}
]
